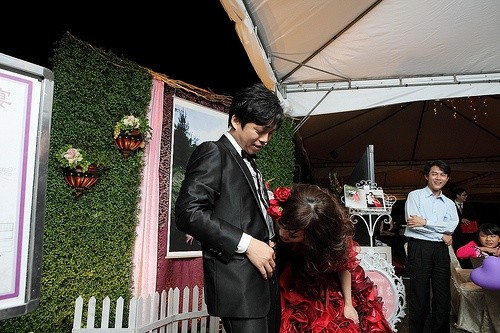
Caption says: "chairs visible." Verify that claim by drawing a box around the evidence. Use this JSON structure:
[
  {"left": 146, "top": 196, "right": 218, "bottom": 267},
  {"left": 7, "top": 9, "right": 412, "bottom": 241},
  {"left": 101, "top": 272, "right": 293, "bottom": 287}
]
[{"left": 448, "top": 245, "right": 500, "bottom": 333}]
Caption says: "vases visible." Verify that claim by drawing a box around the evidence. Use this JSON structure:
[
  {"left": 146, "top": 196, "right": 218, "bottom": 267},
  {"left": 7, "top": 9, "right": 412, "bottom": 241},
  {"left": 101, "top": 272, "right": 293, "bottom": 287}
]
[
  {"left": 63, "top": 164, "right": 103, "bottom": 198},
  {"left": 114, "top": 129, "right": 144, "bottom": 158}
]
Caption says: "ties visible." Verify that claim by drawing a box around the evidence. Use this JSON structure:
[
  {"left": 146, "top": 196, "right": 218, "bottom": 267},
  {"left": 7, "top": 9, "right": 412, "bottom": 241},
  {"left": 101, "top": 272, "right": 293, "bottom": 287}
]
[{"left": 458, "top": 204, "right": 462, "bottom": 208}]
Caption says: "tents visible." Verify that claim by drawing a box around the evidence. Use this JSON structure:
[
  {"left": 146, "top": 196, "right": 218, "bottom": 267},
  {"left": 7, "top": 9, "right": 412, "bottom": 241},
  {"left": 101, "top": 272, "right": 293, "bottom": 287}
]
[{"left": 221, "top": 0, "right": 500, "bottom": 200}]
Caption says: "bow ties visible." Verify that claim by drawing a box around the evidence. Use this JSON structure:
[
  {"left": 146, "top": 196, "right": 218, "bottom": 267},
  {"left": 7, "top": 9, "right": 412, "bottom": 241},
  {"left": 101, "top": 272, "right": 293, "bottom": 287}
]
[{"left": 241, "top": 150, "right": 256, "bottom": 162}]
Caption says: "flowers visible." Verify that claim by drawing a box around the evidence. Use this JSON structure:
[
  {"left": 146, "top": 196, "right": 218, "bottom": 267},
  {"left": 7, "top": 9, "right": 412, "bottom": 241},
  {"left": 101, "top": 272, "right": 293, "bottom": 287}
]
[
  {"left": 264, "top": 180, "right": 274, "bottom": 201},
  {"left": 57, "top": 143, "right": 92, "bottom": 173},
  {"left": 114, "top": 114, "right": 153, "bottom": 139},
  {"left": 268, "top": 186, "right": 292, "bottom": 221}
]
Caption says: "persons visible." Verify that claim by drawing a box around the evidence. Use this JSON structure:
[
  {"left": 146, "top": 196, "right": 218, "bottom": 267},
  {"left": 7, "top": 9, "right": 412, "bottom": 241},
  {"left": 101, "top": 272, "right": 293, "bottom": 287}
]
[
  {"left": 457, "top": 223, "right": 500, "bottom": 259},
  {"left": 450, "top": 187, "right": 471, "bottom": 225},
  {"left": 366, "top": 192, "right": 383, "bottom": 207},
  {"left": 175, "top": 84, "right": 281, "bottom": 333},
  {"left": 347, "top": 189, "right": 360, "bottom": 201},
  {"left": 268, "top": 184, "right": 395, "bottom": 333},
  {"left": 405, "top": 161, "right": 459, "bottom": 333}
]
